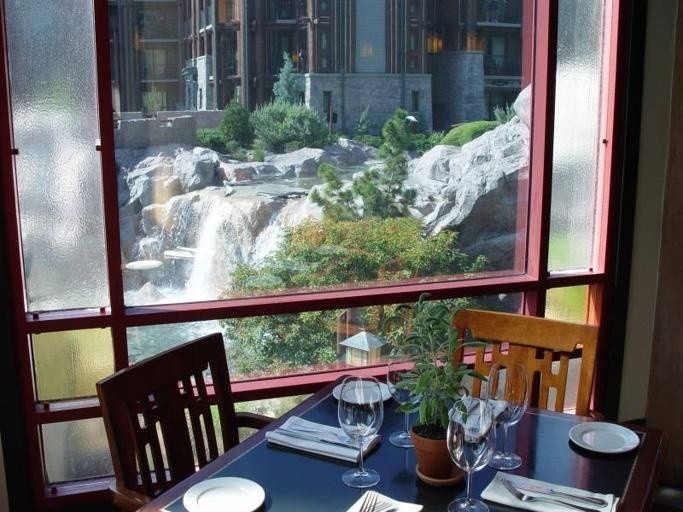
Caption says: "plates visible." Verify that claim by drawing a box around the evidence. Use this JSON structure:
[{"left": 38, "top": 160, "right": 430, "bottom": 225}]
[
  {"left": 181, "top": 477, "right": 265, "bottom": 512},
  {"left": 569, "top": 421, "right": 640, "bottom": 453},
  {"left": 332, "top": 382, "right": 393, "bottom": 405}
]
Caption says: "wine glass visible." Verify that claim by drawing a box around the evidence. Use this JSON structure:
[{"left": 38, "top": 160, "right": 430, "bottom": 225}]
[
  {"left": 488, "top": 361, "right": 528, "bottom": 469},
  {"left": 337, "top": 346, "right": 431, "bottom": 511},
  {"left": 446, "top": 398, "right": 496, "bottom": 512}
]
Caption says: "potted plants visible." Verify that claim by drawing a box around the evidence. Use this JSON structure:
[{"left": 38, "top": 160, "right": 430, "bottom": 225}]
[{"left": 380, "top": 292, "right": 490, "bottom": 486}]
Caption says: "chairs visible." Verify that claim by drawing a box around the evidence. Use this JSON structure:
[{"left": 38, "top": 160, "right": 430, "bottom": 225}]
[
  {"left": 95, "top": 331, "right": 278, "bottom": 512},
  {"left": 452, "top": 308, "right": 606, "bottom": 417}
]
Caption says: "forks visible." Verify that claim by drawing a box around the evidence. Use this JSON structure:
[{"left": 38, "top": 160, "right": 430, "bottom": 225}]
[{"left": 497, "top": 478, "right": 601, "bottom": 512}]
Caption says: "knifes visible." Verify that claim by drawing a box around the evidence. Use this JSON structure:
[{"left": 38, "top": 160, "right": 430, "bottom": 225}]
[
  {"left": 274, "top": 427, "right": 355, "bottom": 451},
  {"left": 513, "top": 480, "right": 606, "bottom": 506}
]
